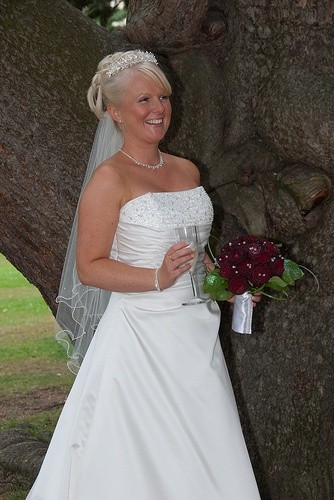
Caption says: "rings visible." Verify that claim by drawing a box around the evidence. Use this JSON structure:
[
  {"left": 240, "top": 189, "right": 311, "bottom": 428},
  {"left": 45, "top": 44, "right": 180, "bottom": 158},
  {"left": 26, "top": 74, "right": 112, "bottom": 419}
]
[{"left": 169, "top": 256, "right": 174, "bottom": 261}]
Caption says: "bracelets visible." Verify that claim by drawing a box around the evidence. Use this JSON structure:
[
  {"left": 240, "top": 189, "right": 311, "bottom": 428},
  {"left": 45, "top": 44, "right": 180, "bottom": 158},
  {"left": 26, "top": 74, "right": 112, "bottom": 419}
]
[{"left": 154, "top": 269, "right": 162, "bottom": 291}]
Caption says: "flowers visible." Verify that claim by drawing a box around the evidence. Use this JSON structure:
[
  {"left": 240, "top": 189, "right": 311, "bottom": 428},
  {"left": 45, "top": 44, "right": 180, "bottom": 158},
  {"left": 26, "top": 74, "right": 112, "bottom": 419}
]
[{"left": 202, "top": 233, "right": 319, "bottom": 335}]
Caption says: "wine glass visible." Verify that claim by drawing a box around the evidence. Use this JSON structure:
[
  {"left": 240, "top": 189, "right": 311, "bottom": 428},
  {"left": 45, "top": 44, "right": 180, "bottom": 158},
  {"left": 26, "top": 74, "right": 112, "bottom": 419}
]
[{"left": 174, "top": 225, "right": 212, "bottom": 306}]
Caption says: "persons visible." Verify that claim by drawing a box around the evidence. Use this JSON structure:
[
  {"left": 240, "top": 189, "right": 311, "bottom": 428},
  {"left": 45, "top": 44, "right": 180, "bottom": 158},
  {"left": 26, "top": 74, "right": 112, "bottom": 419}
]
[{"left": 26, "top": 50, "right": 263, "bottom": 500}]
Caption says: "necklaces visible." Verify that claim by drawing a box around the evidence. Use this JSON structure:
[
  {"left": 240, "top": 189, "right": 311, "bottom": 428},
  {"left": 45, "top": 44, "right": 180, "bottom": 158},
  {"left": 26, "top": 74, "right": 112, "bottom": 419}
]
[{"left": 119, "top": 148, "right": 165, "bottom": 169}]
